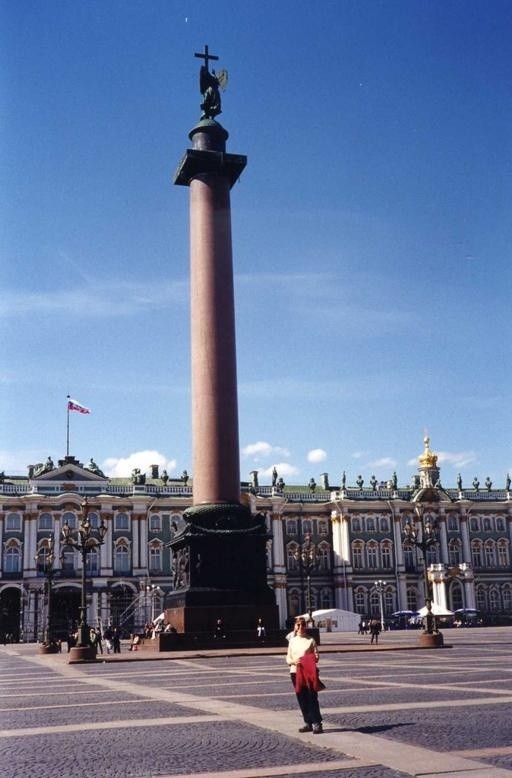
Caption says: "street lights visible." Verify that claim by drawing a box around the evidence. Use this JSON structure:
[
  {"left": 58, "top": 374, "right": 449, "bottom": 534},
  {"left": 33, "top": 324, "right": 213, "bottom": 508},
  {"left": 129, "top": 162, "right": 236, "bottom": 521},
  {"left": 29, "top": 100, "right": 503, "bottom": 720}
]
[
  {"left": 292, "top": 533, "right": 323, "bottom": 630},
  {"left": 374, "top": 578, "right": 388, "bottom": 632},
  {"left": 400, "top": 511, "right": 445, "bottom": 634},
  {"left": 61, "top": 495, "right": 109, "bottom": 647},
  {"left": 31, "top": 533, "right": 66, "bottom": 646},
  {"left": 145, "top": 583, "right": 160, "bottom": 624}
]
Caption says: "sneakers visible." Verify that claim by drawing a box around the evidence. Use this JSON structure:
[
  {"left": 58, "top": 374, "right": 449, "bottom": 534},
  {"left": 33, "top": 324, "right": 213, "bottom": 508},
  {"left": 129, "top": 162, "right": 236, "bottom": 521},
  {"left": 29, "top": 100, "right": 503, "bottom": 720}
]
[
  {"left": 299, "top": 723, "right": 313, "bottom": 732},
  {"left": 312, "top": 723, "right": 322, "bottom": 734}
]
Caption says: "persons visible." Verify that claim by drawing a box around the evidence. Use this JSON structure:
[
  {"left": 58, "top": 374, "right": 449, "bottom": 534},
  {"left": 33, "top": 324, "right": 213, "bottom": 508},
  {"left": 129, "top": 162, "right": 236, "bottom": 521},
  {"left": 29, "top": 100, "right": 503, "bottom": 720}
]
[
  {"left": 256, "top": 618, "right": 268, "bottom": 644},
  {"left": 213, "top": 618, "right": 227, "bottom": 640},
  {"left": 67, "top": 617, "right": 177, "bottom": 656},
  {"left": 368, "top": 619, "right": 380, "bottom": 646},
  {"left": 357, "top": 620, "right": 369, "bottom": 636},
  {"left": 285, "top": 617, "right": 326, "bottom": 734}
]
